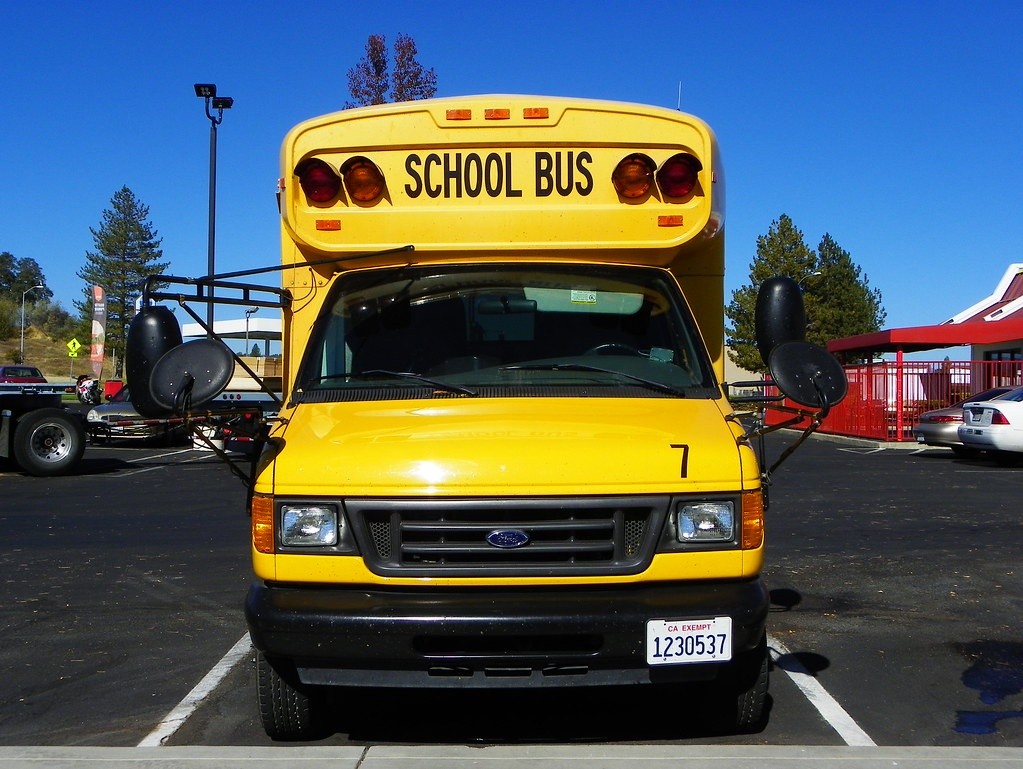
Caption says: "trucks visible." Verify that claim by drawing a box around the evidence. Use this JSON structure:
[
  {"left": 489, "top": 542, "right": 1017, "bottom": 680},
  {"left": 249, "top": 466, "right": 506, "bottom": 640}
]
[{"left": 0, "top": 382, "right": 87, "bottom": 477}]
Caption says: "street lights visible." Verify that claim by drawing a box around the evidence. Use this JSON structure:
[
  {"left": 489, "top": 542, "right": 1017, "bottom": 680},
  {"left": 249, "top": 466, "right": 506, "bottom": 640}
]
[
  {"left": 20, "top": 286, "right": 44, "bottom": 376},
  {"left": 194, "top": 83, "right": 236, "bottom": 339},
  {"left": 244, "top": 306, "right": 260, "bottom": 357}
]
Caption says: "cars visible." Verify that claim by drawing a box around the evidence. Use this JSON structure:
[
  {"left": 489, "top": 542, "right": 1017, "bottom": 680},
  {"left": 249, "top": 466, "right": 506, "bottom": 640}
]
[
  {"left": 87, "top": 382, "right": 189, "bottom": 448},
  {"left": 957, "top": 385, "right": 1023, "bottom": 467},
  {"left": 913, "top": 384, "right": 1023, "bottom": 460},
  {"left": 0, "top": 365, "right": 49, "bottom": 384}
]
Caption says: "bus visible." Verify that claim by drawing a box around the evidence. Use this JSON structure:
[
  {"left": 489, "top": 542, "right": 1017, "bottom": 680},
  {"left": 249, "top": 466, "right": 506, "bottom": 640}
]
[{"left": 123, "top": 94, "right": 849, "bottom": 744}]
